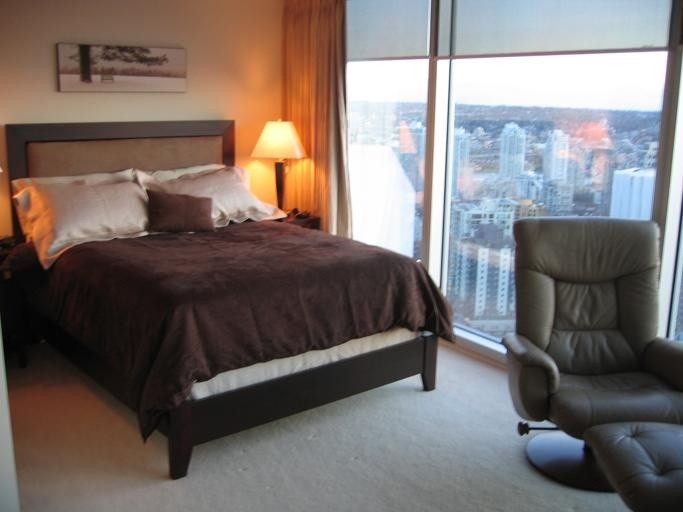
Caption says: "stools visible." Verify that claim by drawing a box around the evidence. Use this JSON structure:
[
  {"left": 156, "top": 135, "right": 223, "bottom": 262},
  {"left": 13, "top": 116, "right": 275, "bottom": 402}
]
[{"left": 583, "top": 421, "right": 683, "bottom": 512}]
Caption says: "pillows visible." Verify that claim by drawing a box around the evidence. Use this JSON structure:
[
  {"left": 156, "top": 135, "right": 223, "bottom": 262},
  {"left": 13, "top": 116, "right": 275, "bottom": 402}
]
[{"left": 10, "top": 163, "right": 290, "bottom": 272}]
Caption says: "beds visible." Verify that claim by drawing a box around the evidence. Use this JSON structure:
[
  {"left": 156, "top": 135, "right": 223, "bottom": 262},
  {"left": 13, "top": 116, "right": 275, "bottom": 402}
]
[{"left": 2, "top": 118, "right": 441, "bottom": 480}]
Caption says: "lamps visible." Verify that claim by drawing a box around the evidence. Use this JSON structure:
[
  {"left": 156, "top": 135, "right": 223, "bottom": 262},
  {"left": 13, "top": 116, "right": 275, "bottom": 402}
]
[{"left": 249, "top": 117, "right": 308, "bottom": 210}]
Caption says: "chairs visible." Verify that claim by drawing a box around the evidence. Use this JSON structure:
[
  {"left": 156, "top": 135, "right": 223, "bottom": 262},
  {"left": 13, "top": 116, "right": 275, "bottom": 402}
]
[{"left": 499, "top": 215, "right": 683, "bottom": 493}]
